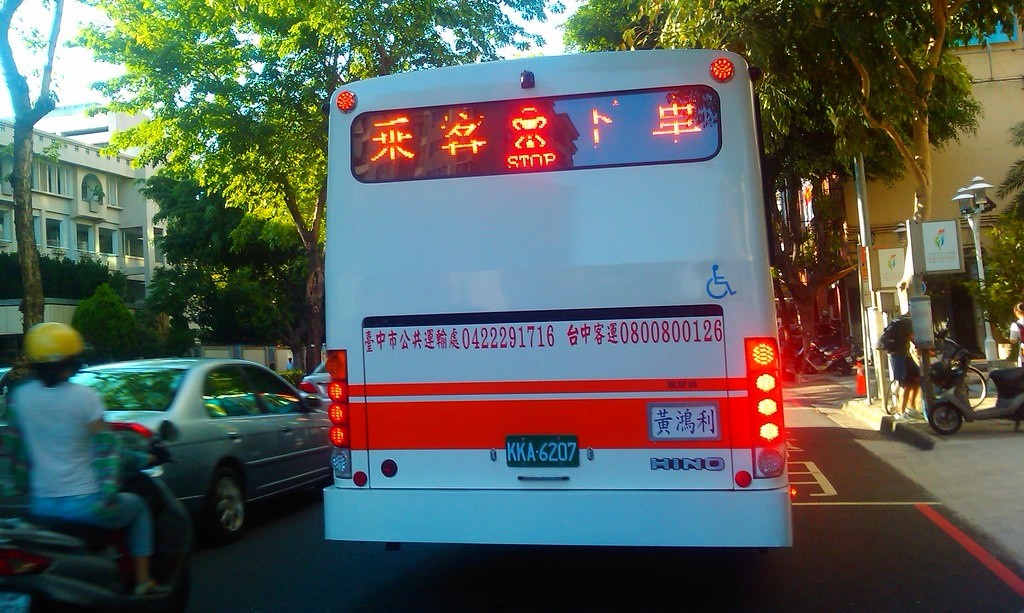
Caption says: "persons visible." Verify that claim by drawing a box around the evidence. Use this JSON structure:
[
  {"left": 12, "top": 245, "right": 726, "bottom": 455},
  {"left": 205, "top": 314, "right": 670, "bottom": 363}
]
[
  {"left": 9, "top": 321, "right": 157, "bottom": 596},
  {"left": 286, "top": 358, "right": 293, "bottom": 370},
  {"left": 1009, "top": 302, "right": 1024, "bottom": 367},
  {"left": 890, "top": 318, "right": 923, "bottom": 421}
]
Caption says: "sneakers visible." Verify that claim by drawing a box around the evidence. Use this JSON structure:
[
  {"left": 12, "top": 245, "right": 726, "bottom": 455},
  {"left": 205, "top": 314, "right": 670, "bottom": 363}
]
[
  {"left": 135, "top": 582, "right": 172, "bottom": 599},
  {"left": 894, "top": 410, "right": 921, "bottom": 420}
]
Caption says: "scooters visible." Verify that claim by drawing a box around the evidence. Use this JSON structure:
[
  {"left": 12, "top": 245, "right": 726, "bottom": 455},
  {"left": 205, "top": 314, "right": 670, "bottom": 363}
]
[
  {"left": 0, "top": 418, "right": 197, "bottom": 613},
  {"left": 922, "top": 328, "right": 1024, "bottom": 436}
]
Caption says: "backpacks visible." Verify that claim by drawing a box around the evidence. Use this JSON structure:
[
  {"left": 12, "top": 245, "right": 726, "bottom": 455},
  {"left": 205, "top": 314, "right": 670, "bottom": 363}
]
[{"left": 881, "top": 319, "right": 906, "bottom": 351}]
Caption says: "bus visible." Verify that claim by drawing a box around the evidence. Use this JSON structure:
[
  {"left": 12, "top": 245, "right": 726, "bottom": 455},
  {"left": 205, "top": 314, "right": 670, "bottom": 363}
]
[{"left": 319, "top": 50, "right": 797, "bottom": 551}]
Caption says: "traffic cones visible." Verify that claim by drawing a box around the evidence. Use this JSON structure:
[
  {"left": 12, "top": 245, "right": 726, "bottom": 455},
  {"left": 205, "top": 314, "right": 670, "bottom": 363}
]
[{"left": 854, "top": 362, "right": 867, "bottom": 399}]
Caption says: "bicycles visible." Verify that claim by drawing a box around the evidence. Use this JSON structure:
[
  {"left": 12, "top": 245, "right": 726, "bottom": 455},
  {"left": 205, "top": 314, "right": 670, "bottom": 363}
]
[{"left": 884, "top": 349, "right": 988, "bottom": 417}]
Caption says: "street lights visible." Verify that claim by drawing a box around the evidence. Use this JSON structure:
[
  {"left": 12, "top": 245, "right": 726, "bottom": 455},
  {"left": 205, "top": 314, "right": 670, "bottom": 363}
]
[{"left": 950, "top": 174, "right": 999, "bottom": 361}]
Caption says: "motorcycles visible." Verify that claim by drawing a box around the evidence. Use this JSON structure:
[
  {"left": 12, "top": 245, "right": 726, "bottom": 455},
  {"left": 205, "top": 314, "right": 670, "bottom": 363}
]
[{"left": 797, "top": 335, "right": 854, "bottom": 378}]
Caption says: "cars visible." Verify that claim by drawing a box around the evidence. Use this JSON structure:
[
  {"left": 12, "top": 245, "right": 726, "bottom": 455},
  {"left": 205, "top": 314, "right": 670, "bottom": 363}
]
[
  {"left": 69, "top": 358, "right": 333, "bottom": 537},
  {"left": 299, "top": 358, "right": 330, "bottom": 398}
]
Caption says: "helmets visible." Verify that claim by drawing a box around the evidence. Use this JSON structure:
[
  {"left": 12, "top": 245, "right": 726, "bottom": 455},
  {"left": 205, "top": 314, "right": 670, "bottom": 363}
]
[{"left": 24, "top": 322, "right": 87, "bottom": 364}]
